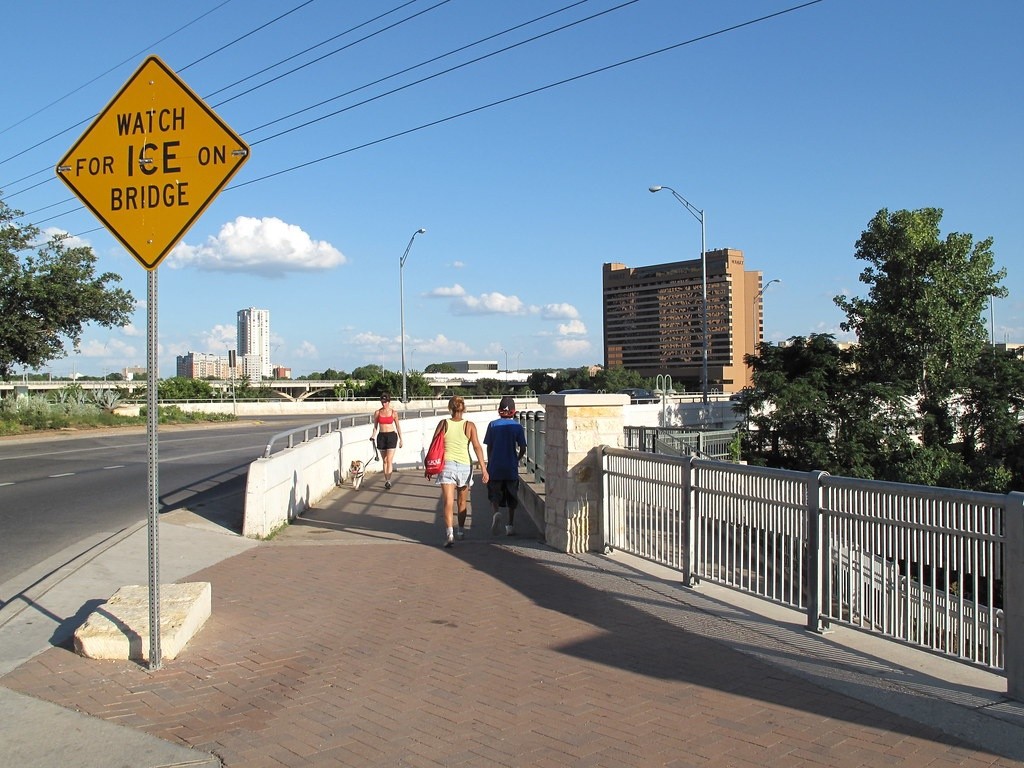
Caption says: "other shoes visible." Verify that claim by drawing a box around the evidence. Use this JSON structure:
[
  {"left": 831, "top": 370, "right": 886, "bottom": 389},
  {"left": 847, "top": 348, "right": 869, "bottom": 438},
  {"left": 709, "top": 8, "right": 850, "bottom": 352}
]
[
  {"left": 457, "top": 532, "right": 464, "bottom": 536},
  {"left": 443, "top": 539, "right": 455, "bottom": 548},
  {"left": 505, "top": 525, "right": 515, "bottom": 536},
  {"left": 385, "top": 481, "right": 392, "bottom": 490},
  {"left": 491, "top": 513, "right": 502, "bottom": 535}
]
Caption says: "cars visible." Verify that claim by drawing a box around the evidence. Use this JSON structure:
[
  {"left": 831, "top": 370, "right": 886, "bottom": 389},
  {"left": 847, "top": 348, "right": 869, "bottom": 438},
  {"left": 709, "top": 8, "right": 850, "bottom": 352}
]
[
  {"left": 617, "top": 387, "right": 661, "bottom": 404},
  {"left": 557, "top": 388, "right": 598, "bottom": 394}
]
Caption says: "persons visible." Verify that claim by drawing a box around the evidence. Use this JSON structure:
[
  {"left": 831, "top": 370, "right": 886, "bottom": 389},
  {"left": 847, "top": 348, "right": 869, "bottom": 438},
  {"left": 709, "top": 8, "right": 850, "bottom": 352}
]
[
  {"left": 483, "top": 397, "right": 527, "bottom": 535},
  {"left": 424, "top": 396, "right": 489, "bottom": 547},
  {"left": 370, "top": 395, "right": 402, "bottom": 488}
]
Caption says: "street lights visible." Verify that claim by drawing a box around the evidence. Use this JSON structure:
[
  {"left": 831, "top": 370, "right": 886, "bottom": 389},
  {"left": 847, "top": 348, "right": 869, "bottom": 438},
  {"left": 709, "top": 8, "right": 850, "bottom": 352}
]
[
  {"left": 399, "top": 227, "right": 425, "bottom": 402},
  {"left": 753, "top": 278, "right": 781, "bottom": 354},
  {"left": 648, "top": 185, "right": 708, "bottom": 404},
  {"left": 501, "top": 348, "right": 508, "bottom": 382}
]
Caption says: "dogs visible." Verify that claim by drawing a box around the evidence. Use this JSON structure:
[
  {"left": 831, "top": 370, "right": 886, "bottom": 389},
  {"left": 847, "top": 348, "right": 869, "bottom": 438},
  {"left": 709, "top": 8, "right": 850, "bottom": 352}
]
[{"left": 349, "top": 461, "right": 367, "bottom": 491}]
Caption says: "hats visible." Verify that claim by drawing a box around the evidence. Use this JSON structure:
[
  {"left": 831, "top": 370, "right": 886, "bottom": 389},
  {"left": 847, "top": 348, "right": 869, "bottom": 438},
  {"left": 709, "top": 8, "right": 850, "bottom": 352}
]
[{"left": 498, "top": 397, "right": 516, "bottom": 417}]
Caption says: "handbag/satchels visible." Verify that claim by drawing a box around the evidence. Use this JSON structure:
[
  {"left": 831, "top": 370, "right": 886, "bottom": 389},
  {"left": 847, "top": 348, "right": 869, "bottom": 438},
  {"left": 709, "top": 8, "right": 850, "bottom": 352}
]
[{"left": 424, "top": 419, "right": 448, "bottom": 475}]
